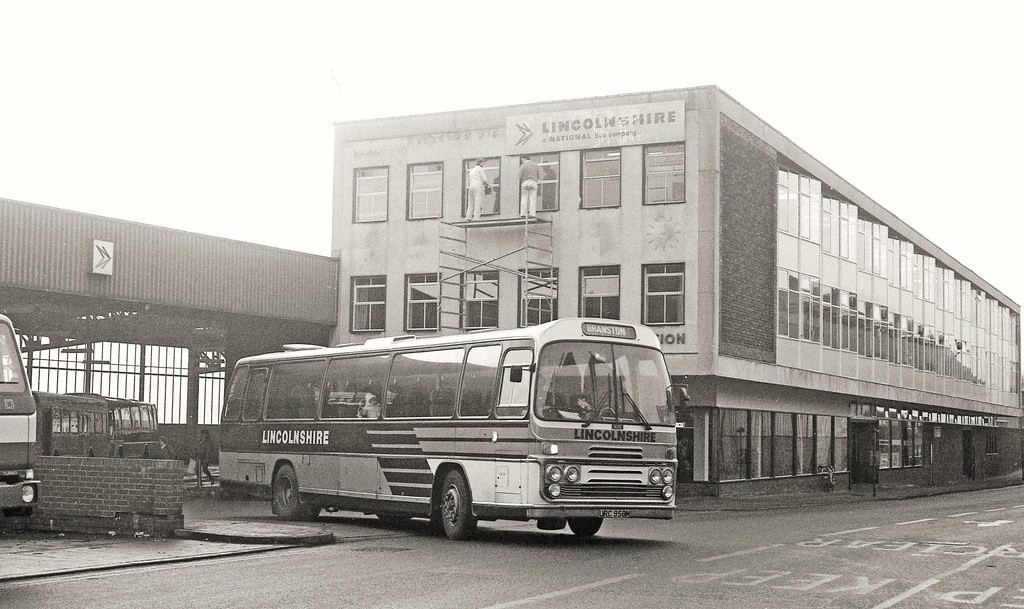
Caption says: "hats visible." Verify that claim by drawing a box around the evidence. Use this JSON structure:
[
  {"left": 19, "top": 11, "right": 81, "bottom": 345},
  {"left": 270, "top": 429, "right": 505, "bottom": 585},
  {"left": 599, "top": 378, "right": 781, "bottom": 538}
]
[{"left": 365, "top": 393, "right": 376, "bottom": 405}]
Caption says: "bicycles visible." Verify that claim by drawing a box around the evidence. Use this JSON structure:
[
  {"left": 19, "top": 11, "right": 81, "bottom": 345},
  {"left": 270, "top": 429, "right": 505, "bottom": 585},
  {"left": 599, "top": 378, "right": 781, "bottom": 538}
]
[{"left": 819, "top": 460, "right": 838, "bottom": 492}]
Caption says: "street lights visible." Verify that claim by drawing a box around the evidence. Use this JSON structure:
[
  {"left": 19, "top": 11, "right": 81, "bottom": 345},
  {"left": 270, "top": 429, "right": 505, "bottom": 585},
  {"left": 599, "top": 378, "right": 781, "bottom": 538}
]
[{"left": 737, "top": 427, "right": 746, "bottom": 479}]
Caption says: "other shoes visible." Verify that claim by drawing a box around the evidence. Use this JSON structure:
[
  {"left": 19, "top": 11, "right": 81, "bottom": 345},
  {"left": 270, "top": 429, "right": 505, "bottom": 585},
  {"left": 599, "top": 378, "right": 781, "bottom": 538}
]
[
  {"left": 210, "top": 478, "right": 214, "bottom": 485},
  {"left": 196, "top": 482, "right": 202, "bottom": 487}
]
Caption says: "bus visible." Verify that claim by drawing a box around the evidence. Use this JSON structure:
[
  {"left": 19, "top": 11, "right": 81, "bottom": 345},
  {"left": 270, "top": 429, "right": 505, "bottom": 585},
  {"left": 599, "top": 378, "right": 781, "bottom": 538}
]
[
  {"left": 0, "top": 312, "right": 40, "bottom": 520},
  {"left": 218, "top": 317, "right": 679, "bottom": 540},
  {"left": 34, "top": 392, "right": 111, "bottom": 459},
  {"left": 105, "top": 395, "right": 163, "bottom": 461}
]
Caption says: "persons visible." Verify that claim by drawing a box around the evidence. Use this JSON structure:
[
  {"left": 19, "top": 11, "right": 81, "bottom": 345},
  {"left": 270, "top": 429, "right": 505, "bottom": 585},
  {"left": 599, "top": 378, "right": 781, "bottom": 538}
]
[
  {"left": 492, "top": 175, "right": 500, "bottom": 214},
  {"left": 517, "top": 154, "right": 540, "bottom": 219},
  {"left": 194, "top": 428, "right": 215, "bottom": 487},
  {"left": 464, "top": 157, "right": 489, "bottom": 221},
  {"left": 356, "top": 392, "right": 383, "bottom": 419},
  {"left": 539, "top": 160, "right": 557, "bottom": 210},
  {"left": 157, "top": 436, "right": 177, "bottom": 460}
]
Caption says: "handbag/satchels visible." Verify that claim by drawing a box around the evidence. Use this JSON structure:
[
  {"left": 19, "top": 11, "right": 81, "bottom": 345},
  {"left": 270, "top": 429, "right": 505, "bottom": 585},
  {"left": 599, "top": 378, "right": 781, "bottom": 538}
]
[{"left": 187, "top": 460, "right": 197, "bottom": 475}]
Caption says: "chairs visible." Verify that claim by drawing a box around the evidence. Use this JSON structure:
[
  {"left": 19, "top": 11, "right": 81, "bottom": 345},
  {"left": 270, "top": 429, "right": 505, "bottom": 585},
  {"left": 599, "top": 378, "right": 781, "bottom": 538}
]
[{"left": 286, "top": 380, "right": 494, "bottom": 417}]
